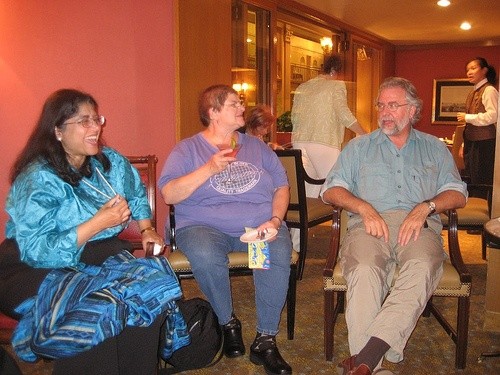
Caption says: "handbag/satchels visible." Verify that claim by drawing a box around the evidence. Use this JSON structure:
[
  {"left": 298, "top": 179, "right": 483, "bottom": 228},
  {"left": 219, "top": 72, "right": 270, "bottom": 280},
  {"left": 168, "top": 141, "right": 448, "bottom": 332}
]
[{"left": 157, "top": 298, "right": 222, "bottom": 375}]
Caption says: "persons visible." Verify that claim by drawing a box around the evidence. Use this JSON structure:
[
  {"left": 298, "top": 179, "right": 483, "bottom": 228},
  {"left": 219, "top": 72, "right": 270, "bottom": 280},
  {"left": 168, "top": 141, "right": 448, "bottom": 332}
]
[
  {"left": 456, "top": 57, "right": 498, "bottom": 234},
  {"left": 157, "top": 84, "right": 292, "bottom": 375},
  {"left": 319, "top": 78, "right": 469, "bottom": 375},
  {"left": 289, "top": 55, "right": 368, "bottom": 253},
  {"left": 0, "top": 88, "right": 191, "bottom": 375},
  {"left": 244, "top": 105, "right": 285, "bottom": 150}
]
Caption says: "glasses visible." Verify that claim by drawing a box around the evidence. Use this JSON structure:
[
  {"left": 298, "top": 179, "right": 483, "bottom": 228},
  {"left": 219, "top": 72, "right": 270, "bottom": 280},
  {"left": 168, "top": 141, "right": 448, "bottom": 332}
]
[
  {"left": 373, "top": 103, "right": 409, "bottom": 112},
  {"left": 222, "top": 102, "right": 243, "bottom": 108},
  {"left": 63, "top": 116, "right": 105, "bottom": 127}
]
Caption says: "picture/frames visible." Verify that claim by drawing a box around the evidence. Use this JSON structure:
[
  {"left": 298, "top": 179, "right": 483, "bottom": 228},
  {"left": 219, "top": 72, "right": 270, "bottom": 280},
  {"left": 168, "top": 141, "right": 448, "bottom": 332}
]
[{"left": 432, "top": 77, "right": 475, "bottom": 126}]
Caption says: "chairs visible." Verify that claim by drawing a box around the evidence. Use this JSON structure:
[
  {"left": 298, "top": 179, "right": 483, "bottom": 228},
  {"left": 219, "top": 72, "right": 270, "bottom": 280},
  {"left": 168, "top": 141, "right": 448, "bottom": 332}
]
[
  {"left": 273, "top": 148, "right": 336, "bottom": 277},
  {"left": 437, "top": 173, "right": 493, "bottom": 260},
  {"left": 114, "top": 154, "right": 156, "bottom": 262},
  {"left": 321, "top": 206, "right": 473, "bottom": 372},
  {"left": 162, "top": 204, "right": 299, "bottom": 338}
]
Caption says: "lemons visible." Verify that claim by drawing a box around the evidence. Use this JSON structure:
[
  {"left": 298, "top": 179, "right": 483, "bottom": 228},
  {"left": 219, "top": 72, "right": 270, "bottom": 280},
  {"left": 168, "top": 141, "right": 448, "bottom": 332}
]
[{"left": 231, "top": 138, "right": 236, "bottom": 150}]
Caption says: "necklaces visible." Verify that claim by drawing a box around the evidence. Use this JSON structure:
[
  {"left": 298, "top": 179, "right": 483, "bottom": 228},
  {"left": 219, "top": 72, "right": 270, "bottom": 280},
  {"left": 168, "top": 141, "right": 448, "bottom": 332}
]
[{"left": 81, "top": 167, "right": 120, "bottom": 203}]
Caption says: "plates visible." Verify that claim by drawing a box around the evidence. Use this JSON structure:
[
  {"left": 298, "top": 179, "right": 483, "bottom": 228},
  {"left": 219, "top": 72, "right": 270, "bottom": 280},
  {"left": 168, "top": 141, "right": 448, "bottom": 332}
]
[{"left": 240, "top": 228, "right": 277, "bottom": 243}]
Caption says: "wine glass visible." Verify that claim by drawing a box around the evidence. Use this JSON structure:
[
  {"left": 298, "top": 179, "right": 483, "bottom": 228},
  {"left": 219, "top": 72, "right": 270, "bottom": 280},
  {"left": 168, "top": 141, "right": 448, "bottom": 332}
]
[{"left": 216, "top": 144, "right": 242, "bottom": 183}]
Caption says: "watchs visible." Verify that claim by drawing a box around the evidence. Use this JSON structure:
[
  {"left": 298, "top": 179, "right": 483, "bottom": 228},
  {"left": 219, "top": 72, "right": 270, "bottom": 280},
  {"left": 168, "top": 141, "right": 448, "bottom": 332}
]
[
  {"left": 423, "top": 200, "right": 436, "bottom": 216},
  {"left": 270, "top": 216, "right": 282, "bottom": 230},
  {"left": 140, "top": 227, "right": 155, "bottom": 233}
]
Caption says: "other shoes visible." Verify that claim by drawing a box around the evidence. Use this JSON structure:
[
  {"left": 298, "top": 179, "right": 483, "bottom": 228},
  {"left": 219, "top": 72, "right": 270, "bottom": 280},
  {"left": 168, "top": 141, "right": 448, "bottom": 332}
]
[{"left": 337, "top": 355, "right": 372, "bottom": 375}]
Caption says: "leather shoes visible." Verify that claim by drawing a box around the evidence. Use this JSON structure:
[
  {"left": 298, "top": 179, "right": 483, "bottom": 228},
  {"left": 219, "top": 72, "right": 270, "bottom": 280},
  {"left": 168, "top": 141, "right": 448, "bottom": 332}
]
[
  {"left": 223, "top": 319, "right": 245, "bottom": 357},
  {"left": 250, "top": 334, "right": 292, "bottom": 375}
]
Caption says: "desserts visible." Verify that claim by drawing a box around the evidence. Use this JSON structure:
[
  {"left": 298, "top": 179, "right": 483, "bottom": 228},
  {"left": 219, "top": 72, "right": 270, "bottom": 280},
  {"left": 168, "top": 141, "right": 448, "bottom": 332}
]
[{"left": 258, "top": 228, "right": 269, "bottom": 240}]
